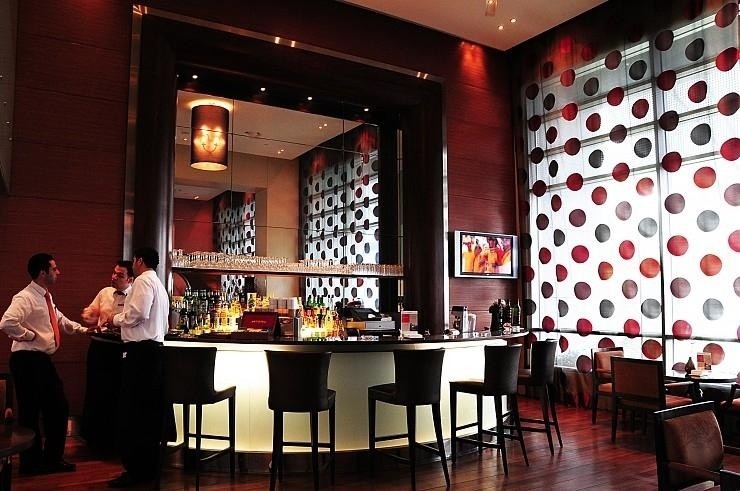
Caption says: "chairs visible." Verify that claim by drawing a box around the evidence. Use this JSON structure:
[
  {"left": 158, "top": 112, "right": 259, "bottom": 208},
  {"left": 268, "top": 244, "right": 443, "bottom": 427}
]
[
  {"left": 608, "top": 355, "right": 694, "bottom": 444},
  {"left": 158, "top": 344, "right": 238, "bottom": 491},
  {"left": 496, "top": 338, "right": 565, "bottom": 457},
  {"left": 716, "top": 384, "right": 740, "bottom": 432},
  {"left": 449, "top": 343, "right": 530, "bottom": 479},
  {"left": 590, "top": 347, "right": 625, "bottom": 426},
  {"left": 0, "top": 374, "right": 15, "bottom": 473},
  {"left": 366, "top": 347, "right": 451, "bottom": 491},
  {"left": 263, "top": 349, "right": 337, "bottom": 491},
  {"left": 651, "top": 400, "right": 739, "bottom": 491}
]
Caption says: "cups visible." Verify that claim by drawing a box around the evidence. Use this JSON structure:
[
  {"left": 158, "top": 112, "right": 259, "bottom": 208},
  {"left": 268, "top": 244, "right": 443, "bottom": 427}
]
[
  {"left": 268, "top": 297, "right": 298, "bottom": 309},
  {"left": 246, "top": 293, "right": 257, "bottom": 311},
  {"left": 691, "top": 370, "right": 702, "bottom": 375}
]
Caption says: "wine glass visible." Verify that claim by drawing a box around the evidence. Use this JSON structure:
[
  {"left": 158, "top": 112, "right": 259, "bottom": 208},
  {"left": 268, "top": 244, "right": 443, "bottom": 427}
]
[{"left": 170, "top": 248, "right": 403, "bottom": 275}]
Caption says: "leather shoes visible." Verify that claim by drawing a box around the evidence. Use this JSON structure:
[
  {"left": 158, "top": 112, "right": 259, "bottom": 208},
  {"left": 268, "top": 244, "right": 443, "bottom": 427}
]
[{"left": 41, "top": 461, "right": 77, "bottom": 472}]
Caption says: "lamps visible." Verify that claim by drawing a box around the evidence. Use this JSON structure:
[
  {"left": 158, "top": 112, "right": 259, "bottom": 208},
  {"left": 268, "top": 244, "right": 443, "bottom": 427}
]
[{"left": 188, "top": 103, "right": 230, "bottom": 173}]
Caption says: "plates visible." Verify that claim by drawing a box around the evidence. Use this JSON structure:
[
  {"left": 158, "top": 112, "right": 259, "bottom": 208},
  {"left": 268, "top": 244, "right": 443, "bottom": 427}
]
[{"left": 689, "top": 374, "right": 700, "bottom": 377}]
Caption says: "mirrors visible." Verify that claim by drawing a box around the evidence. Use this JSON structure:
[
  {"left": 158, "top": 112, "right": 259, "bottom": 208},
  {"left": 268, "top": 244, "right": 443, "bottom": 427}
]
[{"left": 170, "top": 84, "right": 404, "bottom": 312}]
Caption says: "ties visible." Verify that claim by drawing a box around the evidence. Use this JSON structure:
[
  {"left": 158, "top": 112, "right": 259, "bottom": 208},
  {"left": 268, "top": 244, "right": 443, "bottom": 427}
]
[{"left": 45, "top": 292, "right": 60, "bottom": 347}]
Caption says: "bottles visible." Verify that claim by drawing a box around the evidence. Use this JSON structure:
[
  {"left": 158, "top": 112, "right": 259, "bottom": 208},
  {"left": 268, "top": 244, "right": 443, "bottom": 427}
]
[
  {"left": 685, "top": 356, "right": 695, "bottom": 373},
  {"left": 496, "top": 297, "right": 521, "bottom": 329},
  {"left": 179, "top": 288, "right": 241, "bottom": 334},
  {"left": 295, "top": 294, "right": 344, "bottom": 342}
]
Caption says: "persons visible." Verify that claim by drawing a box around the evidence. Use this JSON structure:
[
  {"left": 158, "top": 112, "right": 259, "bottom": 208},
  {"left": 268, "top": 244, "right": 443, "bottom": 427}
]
[
  {"left": 81, "top": 260, "right": 135, "bottom": 458},
  {"left": 462, "top": 235, "right": 512, "bottom": 275},
  {"left": 0, "top": 253, "right": 100, "bottom": 477},
  {"left": 100, "top": 246, "right": 169, "bottom": 490}
]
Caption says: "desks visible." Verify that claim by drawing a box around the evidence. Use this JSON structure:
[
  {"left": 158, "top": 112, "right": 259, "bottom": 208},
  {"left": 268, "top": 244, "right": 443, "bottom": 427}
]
[
  {"left": 664, "top": 370, "right": 737, "bottom": 398},
  {"left": 0, "top": 421, "right": 37, "bottom": 491}
]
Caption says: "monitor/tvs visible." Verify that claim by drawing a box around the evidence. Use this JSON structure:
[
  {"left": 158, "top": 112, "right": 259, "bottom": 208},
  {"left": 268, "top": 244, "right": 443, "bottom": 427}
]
[
  {"left": 454, "top": 230, "right": 518, "bottom": 279},
  {"left": 350, "top": 308, "right": 382, "bottom": 321}
]
